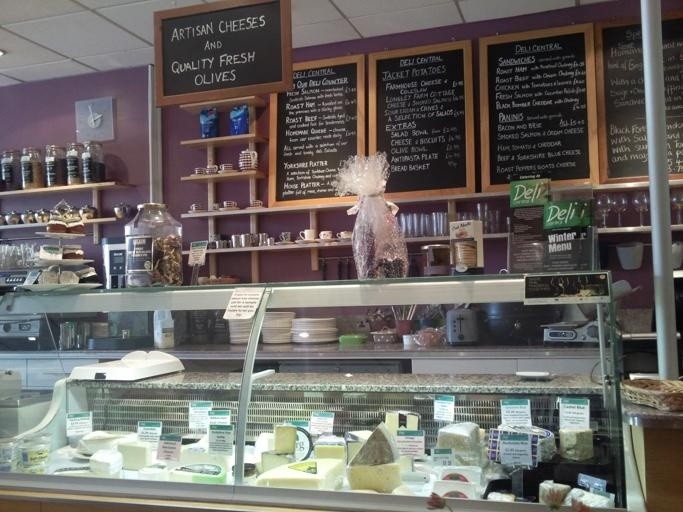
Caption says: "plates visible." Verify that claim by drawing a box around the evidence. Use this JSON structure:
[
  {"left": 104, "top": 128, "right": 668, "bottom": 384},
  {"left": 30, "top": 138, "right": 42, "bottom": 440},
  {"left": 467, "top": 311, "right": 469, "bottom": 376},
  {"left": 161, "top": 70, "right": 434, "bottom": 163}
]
[{"left": 226, "top": 311, "right": 339, "bottom": 345}]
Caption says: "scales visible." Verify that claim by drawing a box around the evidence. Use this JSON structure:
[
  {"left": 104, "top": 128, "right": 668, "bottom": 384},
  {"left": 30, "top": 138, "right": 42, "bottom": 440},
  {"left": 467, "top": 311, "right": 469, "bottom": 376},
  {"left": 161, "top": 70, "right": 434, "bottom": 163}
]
[{"left": 0, "top": 263, "right": 96, "bottom": 287}]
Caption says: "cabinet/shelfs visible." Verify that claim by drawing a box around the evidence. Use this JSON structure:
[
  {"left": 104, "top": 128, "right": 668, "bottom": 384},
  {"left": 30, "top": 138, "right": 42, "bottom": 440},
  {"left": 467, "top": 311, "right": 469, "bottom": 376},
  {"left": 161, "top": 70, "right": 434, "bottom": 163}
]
[
  {"left": 172, "top": 176, "right": 682, "bottom": 255},
  {"left": 176, "top": 93, "right": 268, "bottom": 185},
  {"left": 1, "top": 180, "right": 136, "bottom": 230}
]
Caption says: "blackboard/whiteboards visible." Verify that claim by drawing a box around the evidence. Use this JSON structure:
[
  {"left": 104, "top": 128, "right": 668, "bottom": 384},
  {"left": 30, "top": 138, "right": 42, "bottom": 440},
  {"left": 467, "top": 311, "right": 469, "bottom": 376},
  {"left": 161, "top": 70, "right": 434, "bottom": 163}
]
[
  {"left": 268, "top": 53, "right": 366, "bottom": 210},
  {"left": 150, "top": 0, "right": 293, "bottom": 108},
  {"left": 479, "top": 23, "right": 599, "bottom": 193},
  {"left": 368, "top": 40, "right": 476, "bottom": 200},
  {"left": 595, "top": 13, "right": 683, "bottom": 184}
]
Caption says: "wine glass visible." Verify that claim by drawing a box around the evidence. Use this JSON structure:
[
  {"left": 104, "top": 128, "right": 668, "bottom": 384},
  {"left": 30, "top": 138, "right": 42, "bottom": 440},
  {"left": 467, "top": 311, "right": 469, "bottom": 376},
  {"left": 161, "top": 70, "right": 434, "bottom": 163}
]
[{"left": 596, "top": 190, "right": 682, "bottom": 228}]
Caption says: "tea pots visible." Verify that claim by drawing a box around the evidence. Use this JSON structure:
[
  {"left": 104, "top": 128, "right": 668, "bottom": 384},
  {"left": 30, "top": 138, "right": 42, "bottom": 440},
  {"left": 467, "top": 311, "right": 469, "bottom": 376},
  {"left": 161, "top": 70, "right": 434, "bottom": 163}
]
[
  {"left": 0, "top": 201, "right": 130, "bottom": 225},
  {"left": 238, "top": 149, "right": 258, "bottom": 171}
]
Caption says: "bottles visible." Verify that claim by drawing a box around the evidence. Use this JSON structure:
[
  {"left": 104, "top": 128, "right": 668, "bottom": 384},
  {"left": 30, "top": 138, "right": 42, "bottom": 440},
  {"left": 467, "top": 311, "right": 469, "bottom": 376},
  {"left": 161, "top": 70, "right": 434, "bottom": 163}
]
[
  {"left": 152, "top": 310, "right": 174, "bottom": 348},
  {"left": 0, "top": 140, "right": 105, "bottom": 191}
]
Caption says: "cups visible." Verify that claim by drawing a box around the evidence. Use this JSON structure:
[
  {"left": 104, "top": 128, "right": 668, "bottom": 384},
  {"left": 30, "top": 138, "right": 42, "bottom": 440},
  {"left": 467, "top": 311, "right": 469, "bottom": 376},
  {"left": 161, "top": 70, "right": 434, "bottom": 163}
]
[
  {"left": 213, "top": 229, "right": 351, "bottom": 250},
  {"left": 397, "top": 202, "right": 500, "bottom": 237},
  {"left": 402, "top": 334, "right": 416, "bottom": 353},
  {"left": 189, "top": 163, "right": 264, "bottom": 212},
  {"left": 394, "top": 319, "right": 412, "bottom": 341},
  {"left": 614, "top": 242, "right": 644, "bottom": 271}
]
